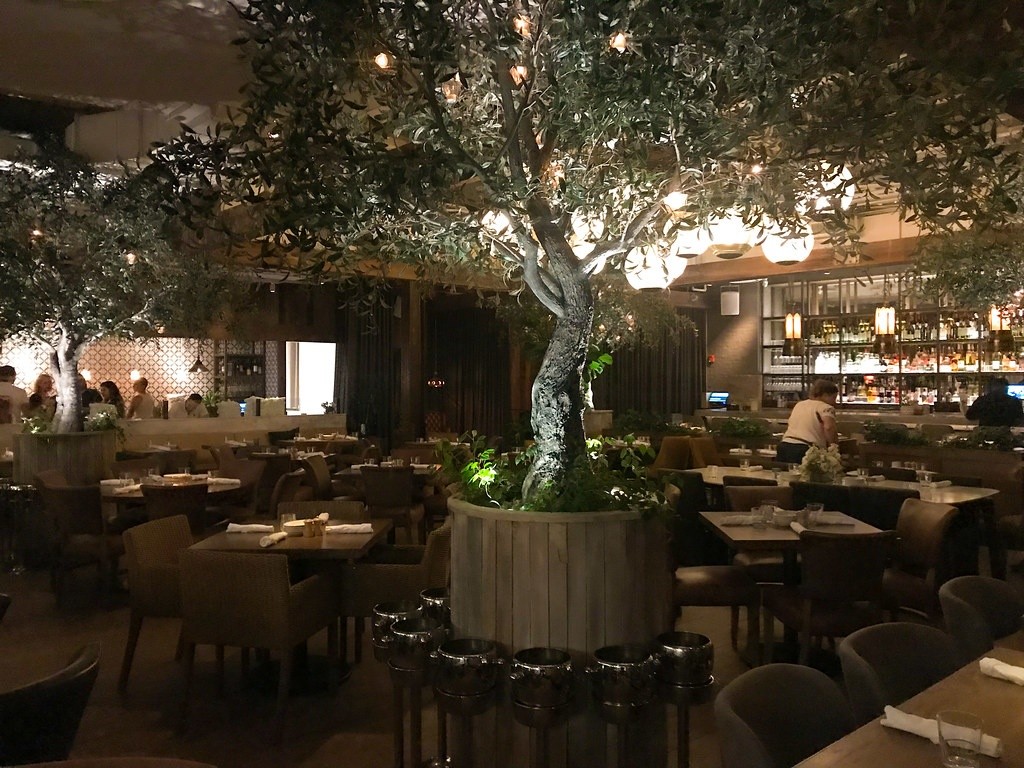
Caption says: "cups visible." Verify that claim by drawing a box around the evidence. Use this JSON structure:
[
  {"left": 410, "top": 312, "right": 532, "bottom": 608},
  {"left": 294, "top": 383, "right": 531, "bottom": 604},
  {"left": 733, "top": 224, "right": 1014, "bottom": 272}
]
[
  {"left": 858, "top": 469, "right": 868, "bottom": 480},
  {"left": 937, "top": 710, "right": 983, "bottom": 768},
  {"left": 740, "top": 459, "right": 749, "bottom": 471},
  {"left": 752, "top": 504, "right": 774, "bottom": 528},
  {"left": 771, "top": 365, "right": 808, "bottom": 374},
  {"left": 119, "top": 473, "right": 130, "bottom": 487},
  {"left": 769, "top": 445, "right": 777, "bottom": 456},
  {"left": 739, "top": 445, "right": 745, "bottom": 454},
  {"left": 772, "top": 468, "right": 781, "bottom": 484},
  {"left": 708, "top": 466, "right": 717, "bottom": 478},
  {"left": 281, "top": 514, "right": 295, "bottom": 532},
  {"left": 807, "top": 503, "right": 823, "bottom": 525},
  {"left": 919, "top": 473, "right": 931, "bottom": 486}
]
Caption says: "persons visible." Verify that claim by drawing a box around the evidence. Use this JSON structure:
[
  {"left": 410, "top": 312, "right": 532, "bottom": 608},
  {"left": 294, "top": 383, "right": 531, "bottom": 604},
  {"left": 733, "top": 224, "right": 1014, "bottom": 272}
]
[
  {"left": 128, "top": 377, "right": 154, "bottom": 418},
  {"left": 101, "top": 381, "right": 125, "bottom": 418},
  {"left": 966, "top": 379, "right": 1024, "bottom": 427},
  {"left": 0, "top": 365, "right": 37, "bottom": 424},
  {"left": 29, "top": 374, "right": 57, "bottom": 412},
  {"left": 188, "top": 394, "right": 207, "bottom": 418},
  {"left": 775, "top": 379, "right": 839, "bottom": 464},
  {"left": 78, "top": 373, "right": 101, "bottom": 408}
]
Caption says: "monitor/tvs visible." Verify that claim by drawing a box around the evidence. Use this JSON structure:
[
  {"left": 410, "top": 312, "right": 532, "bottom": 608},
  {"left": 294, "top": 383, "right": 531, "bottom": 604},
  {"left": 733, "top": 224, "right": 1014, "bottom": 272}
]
[
  {"left": 708, "top": 391, "right": 729, "bottom": 408},
  {"left": 239, "top": 403, "right": 246, "bottom": 414},
  {"left": 1007, "top": 384, "right": 1024, "bottom": 401}
]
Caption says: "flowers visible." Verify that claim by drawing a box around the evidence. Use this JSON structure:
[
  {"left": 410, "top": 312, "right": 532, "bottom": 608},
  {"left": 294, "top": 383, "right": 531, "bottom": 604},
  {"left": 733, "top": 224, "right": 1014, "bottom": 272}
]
[{"left": 802, "top": 444, "right": 841, "bottom": 472}]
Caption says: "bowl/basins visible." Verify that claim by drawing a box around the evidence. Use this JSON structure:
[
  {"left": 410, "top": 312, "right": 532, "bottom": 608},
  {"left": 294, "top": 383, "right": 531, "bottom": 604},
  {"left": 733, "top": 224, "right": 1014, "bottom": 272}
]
[
  {"left": 284, "top": 518, "right": 328, "bottom": 536},
  {"left": 773, "top": 511, "right": 811, "bottom": 527},
  {"left": 781, "top": 472, "right": 802, "bottom": 482}
]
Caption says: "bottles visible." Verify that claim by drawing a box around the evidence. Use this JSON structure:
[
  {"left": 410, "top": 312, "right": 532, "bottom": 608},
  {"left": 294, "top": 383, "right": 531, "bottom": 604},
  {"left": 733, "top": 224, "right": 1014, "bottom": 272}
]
[
  {"left": 810, "top": 321, "right": 840, "bottom": 374},
  {"left": 982, "top": 350, "right": 1024, "bottom": 371},
  {"left": 939, "top": 314, "right": 988, "bottom": 340},
  {"left": 901, "top": 314, "right": 937, "bottom": 405},
  {"left": 939, "top": 343, "right": 979, "bottom": 406},
  {"left": 842, "top": 319, "right": 899, "bottom": 404}
]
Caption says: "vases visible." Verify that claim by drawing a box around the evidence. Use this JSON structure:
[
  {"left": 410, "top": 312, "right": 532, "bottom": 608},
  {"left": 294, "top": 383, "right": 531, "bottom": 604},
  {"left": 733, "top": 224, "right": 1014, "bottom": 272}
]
[{"left": 811, "top": 471, "right": 834, "bottom": 482}]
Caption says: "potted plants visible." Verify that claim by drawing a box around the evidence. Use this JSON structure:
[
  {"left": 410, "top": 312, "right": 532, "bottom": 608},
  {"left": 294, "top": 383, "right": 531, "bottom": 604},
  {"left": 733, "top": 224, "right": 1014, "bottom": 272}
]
[{"left": 203, "top": 391, "right": 219, "bottom": 417}]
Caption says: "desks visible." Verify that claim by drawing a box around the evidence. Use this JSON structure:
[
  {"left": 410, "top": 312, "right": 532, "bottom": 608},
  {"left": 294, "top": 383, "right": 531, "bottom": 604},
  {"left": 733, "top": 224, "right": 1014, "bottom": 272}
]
[
  {"left": 336, "top": 464, "right": 442, "bottom": 544},
  {"left": 277, "top": 439, "right": 359, "bottom": 454},
  {"left": 0, "top": 414, "right": 346, "bottom": 475},
  {"left": 688, "top": 467, "right": 1000, "bottom": 546},
  {"left": 193, "top": 518, "right": 393, "bottom": 674},
  {"left": 100, "top": 477, "right": 243, "bottom": 570},
  {"left": 699, "top": 512, "right": 883, "bottom": 668},
  {"left": 794, "top": 647, "right": 1024, "bottom": 768}
]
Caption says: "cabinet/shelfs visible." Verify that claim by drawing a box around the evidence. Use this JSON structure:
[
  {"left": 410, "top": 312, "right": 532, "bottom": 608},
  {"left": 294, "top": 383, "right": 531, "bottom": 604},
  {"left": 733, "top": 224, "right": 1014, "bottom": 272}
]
[
  {"left": 762, "top": 254, "right": 1024, "bottom": 412},
  {"left": 213, "top": 339, "right": 265, "bottom": 401}
]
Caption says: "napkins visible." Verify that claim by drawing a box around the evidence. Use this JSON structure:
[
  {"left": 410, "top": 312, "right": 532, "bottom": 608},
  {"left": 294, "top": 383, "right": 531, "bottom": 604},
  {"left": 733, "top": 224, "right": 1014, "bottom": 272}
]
[
  {"left": 721, "top": 515, "right": 754, "bottom": 525},
  {"left": 319, "top": 512, "right": 329, "bottom": 523},
  {"left": 790, "top": 522, "right": 807, "bottom": 534},
  {"left": 979, "top": 656, "right": 1024, "bottom": 686},
  {"left": 263, "top": 531, "right": 288, "bottom": 544},
  {"left": 207, "top": 477, "right": 240, "bottom": 484},
  {"left": 931, "top": 480, "right": 952, "bottom": 488},
  {"left": 100, "top": 479, "right": 134, "bottom": 485},
  {"left": 881, "top": 705, "right": 1002, "bottom": 757},
  {"left": 750, "top": 465, "right": 763, "bottom": 471},
  {"left": 226, "top": 523, "right": 274, "bottom": 532},
  {"left": 326, "top": 523, "right": 373, "bottom": 533},
  {"left": 819, "top": 516, "right": 855, "bottom": 525},
  {"left": 113, "top": 484, "right": 142, "bottom": 494}
]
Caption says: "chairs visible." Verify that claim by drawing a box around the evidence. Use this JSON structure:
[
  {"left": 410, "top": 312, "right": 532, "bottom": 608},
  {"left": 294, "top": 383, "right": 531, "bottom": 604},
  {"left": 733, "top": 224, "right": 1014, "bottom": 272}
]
[{"left": 0, "top": 416, "right": 1024, "bottom": 768}]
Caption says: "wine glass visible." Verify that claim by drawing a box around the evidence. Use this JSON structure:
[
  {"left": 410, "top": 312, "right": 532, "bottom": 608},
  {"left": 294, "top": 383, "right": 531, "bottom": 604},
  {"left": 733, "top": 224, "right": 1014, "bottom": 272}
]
[
  {"left": 772, "top": 349, "right": 812, "bottom": 364},
  {"left": 764, "top": 377, "right": 807, "bottom": 390}
]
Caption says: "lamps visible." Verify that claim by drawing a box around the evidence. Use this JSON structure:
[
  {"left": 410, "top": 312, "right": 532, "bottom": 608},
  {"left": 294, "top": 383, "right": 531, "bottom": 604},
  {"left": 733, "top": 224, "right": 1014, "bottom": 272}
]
[
  {"left": 189, "top": 340, "right": 209, "bottom": 372},
  {"left": 270, "top": 283, "right": 275, "bottom": 292},
  {"left": 427, "top": 319, "right": 447, "bottom": 387},
  {"left": 784, "top": 274, "right": 801, "bottom": 339},
  {"left": 874, "top": 273, "right": 895, "bottom": 335}
]
